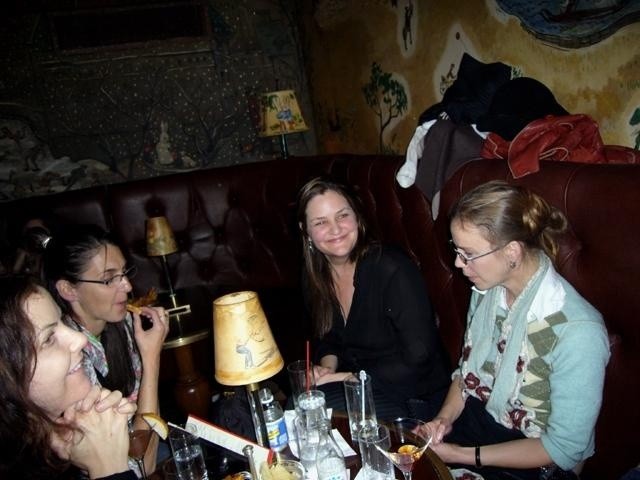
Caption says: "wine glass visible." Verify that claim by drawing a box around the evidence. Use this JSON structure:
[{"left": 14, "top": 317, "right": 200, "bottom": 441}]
[
  {"left": 127, "top": 419, "right": 157, "bottom": 479},
  {"left": 372, "top": 417, "right": 432, "bottom": 480}
]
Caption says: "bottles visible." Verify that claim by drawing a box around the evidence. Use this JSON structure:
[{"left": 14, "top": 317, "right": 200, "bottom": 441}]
[
  {"left": 315, "top": 418, "right": 347, "bottom": 480},
  {"left": 242, "top": 445, "right": 259, "bottom": 480},
  {"left": 258, "top": 387, "right": 300, "bottom": 460}
]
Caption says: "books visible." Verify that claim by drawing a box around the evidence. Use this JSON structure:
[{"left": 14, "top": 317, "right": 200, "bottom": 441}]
[{"left": 163, "top": 414, "right": 274, "bottom": 479}]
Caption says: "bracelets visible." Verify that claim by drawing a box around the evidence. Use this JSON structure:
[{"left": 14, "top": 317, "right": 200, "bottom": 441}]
[{"left": 474, "top": 445, "right": 482, "bottom": 468}]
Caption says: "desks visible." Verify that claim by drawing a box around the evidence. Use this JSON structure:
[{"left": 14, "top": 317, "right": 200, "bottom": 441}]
[
  {"left": 146, "top": 408, "right": 452, "bottom": 480},
  {"left": 145, "top": 284, "right": 234, "bottom": 416}
]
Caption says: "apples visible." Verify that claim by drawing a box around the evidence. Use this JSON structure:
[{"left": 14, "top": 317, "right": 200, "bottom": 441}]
[{"left": 141, "top": 412, "right": 169, "bottom": 440}]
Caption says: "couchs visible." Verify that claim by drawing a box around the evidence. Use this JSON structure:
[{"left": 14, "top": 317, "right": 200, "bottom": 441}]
[{"left": 0, "top": 153, "right": 640, "bottom": 480}]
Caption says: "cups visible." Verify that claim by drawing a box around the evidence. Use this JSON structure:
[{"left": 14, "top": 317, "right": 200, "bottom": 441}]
[
  {"left": 168, "top": 423, "right": 209, "bottom": 480},
  {"left": 344, "top": 374, "right": 396, "bottom": 480},
  {"left": 287, "top": 360, "right": 331, "bottom": 473},
  {"left": 260, "top": 460, "right": 306, "bottom": 480}
]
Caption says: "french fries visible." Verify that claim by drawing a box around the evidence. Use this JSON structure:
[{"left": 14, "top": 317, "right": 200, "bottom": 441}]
[{"left": 125, "top": 303, "right": 141, "bottom": 315}]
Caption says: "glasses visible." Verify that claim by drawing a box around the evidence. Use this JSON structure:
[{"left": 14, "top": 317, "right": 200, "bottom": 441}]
[
  {"left": 449, "top": 240, "right": 502, "bottom": 265},
  {"left": 78, "top": 263, "right": 139, "bottom": 289}
]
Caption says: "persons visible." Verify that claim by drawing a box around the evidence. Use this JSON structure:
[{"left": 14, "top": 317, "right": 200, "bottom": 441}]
[
  {"left": 9, "top": 221, "right": 175, "bottom": 478},
  {"left": 0, "top": 264, "right": 144, "bottom": 478},
  {"left": 410, "top": 177, "right": 613, "bottom": 480},
  {"left": 281, "top": 176, "right": 459, "bottom": 430}
]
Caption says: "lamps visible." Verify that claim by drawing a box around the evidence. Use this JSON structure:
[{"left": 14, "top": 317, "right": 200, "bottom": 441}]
[
  {"left": 257, "top": 89, "right": 309, "bottom": 159},
  {"left": 213, "top": 291, "right": 284, "bottom": 449},
  {"left": 144, "top": 216, "right": 191, "bottom": 314}
]
